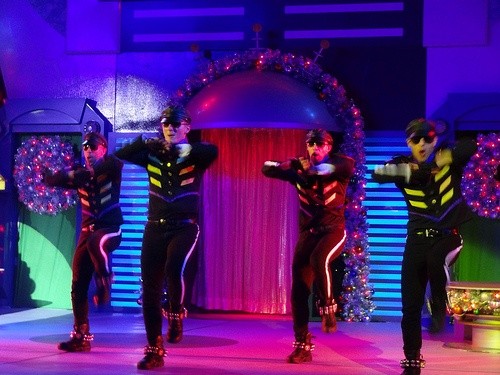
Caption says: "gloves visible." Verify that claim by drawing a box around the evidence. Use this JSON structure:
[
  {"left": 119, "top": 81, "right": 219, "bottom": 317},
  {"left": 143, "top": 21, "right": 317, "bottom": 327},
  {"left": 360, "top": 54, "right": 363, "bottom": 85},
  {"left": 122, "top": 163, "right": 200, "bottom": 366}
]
[
  {"left": 172, "top": 144, "right": 192, "bottom": 164},
  {"left": 145, "top": 137, "right": 171, "bottom": 153}
]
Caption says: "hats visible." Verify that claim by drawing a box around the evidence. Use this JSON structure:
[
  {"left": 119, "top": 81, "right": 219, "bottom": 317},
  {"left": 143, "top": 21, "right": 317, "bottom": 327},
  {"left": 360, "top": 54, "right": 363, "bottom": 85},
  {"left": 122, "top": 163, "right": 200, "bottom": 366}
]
[
  {"left": 305, "top": 128, "right": 334, "bottom": 144},
  {"left": 406, "top": 119, "right": 440, "bottom": 140},
  {"left": 82, "top": 132, "right": 108, "bottom": 148},
  {"left": 160, "top": 105, "right": 191, "bottom": 123}
]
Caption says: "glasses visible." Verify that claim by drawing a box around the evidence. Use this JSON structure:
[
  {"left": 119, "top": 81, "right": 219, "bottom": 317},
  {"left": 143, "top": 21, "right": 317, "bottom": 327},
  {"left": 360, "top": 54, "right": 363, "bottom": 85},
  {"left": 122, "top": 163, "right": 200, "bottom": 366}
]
[
  {"left": 308, "top": 141, "right": 330, "bottom": 147},
  {"left": 161, "top": 122, "right": 188, "bottom": 129},
  {"left": 83, "top": 143, "right": 98, "bottom": 151},
  {"left": 407, "top": 134, "right": 436, "bottom": 144}
]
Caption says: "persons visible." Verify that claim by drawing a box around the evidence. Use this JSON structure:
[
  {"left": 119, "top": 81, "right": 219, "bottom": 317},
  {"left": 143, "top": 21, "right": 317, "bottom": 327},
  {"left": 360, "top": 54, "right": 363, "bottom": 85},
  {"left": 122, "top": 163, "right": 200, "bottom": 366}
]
[
  {"left": 261, "top": 128, "right": 355, "bottom": 363},
  {"left": 113, "top": 106, "right": 218, "bottom": 369},
  {"left": 372, "top": 118, "right": 476, "bottom": 375},
  {"left": 46, "top": 132, "right": 124, "bottom": 353}
]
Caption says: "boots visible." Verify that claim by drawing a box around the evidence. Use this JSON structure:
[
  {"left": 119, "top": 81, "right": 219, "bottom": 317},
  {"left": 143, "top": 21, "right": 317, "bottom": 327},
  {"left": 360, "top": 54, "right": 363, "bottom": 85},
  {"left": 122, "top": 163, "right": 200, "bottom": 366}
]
[
  {"left": 57, "top": 323, "right": 94, "bottom": 352},
  {"left": 136, "top": 335, "right": 168, "bottom": 369},
  {"left": 287, "top": 332, "right": 317, "bottom": 364},
  {"left": 161, "top": 301, "right": 188, "bottom": 343},
  {"left": 316, "top": 297, "right": 338, "bottom": 333},
  {"left": 94, "top": 272, "right": 115, "bottom": 307},
  {"left": 399, "top": 346, "right": 426, "bottom": 375}
]
